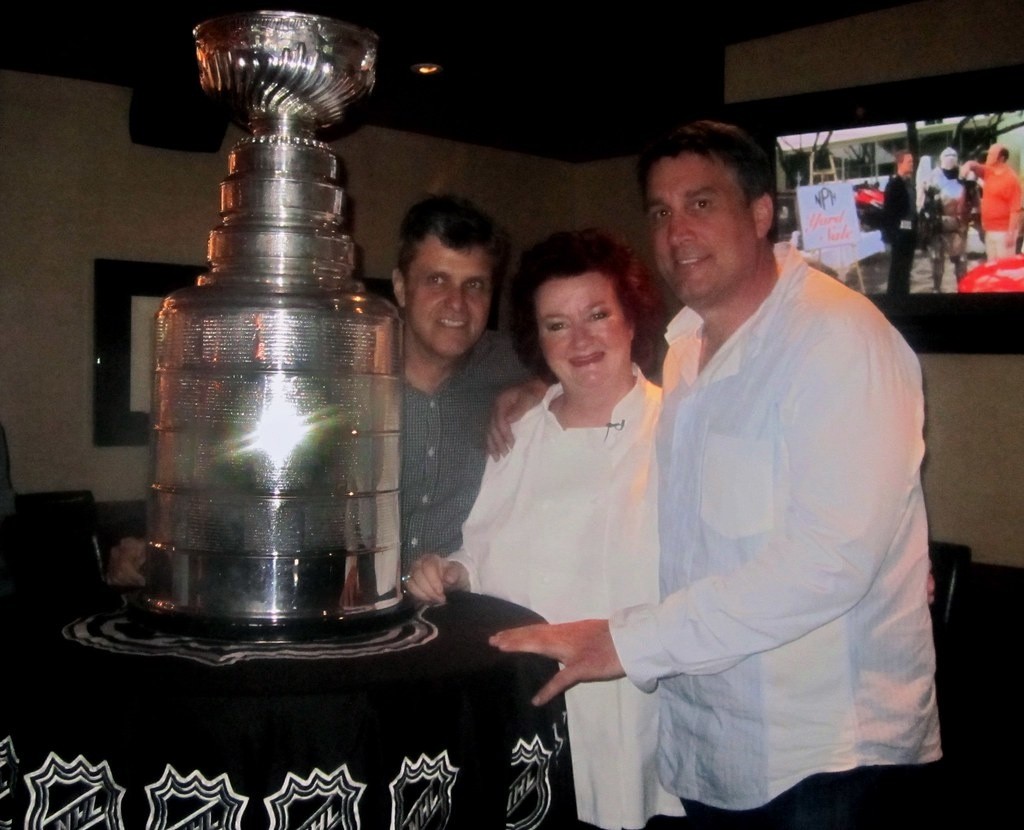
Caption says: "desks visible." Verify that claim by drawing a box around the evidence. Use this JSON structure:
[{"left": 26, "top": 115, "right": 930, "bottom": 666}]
[{"left": 0, "top": 582, "right": 585, "bottom": 830}]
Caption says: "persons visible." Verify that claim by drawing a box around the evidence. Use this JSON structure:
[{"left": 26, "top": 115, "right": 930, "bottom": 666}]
[
  {"left": 392, "top": 191, "right": 547, "bottom": 593},
  {"left": 487, "top": 120, "right": 944, "bottom": 829},
  {"left": 882, "top": 142, "right": 1024, "bottom": 294},
  {"left": 404, "top": 225, "right": 936, "bottom": 830}
]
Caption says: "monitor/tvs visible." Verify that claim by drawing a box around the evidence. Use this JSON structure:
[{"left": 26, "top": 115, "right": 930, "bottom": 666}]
[{"left": 714, "top": 65, "right": 1024, "bottom": 355}]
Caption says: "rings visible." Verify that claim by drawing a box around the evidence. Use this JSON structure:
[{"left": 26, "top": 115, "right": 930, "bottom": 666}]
[{"left": 400, "top": 575, "right": 412, "bottom": 581}]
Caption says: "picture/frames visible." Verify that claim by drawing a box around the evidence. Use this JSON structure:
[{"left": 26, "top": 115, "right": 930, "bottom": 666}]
[{"left": 93, "top": 258, "right": 500, "bottom": 447}]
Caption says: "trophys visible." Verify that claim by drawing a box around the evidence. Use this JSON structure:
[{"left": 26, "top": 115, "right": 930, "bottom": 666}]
[{"left": 127, "top": 10, "right": 406, "bottom": 624}]
